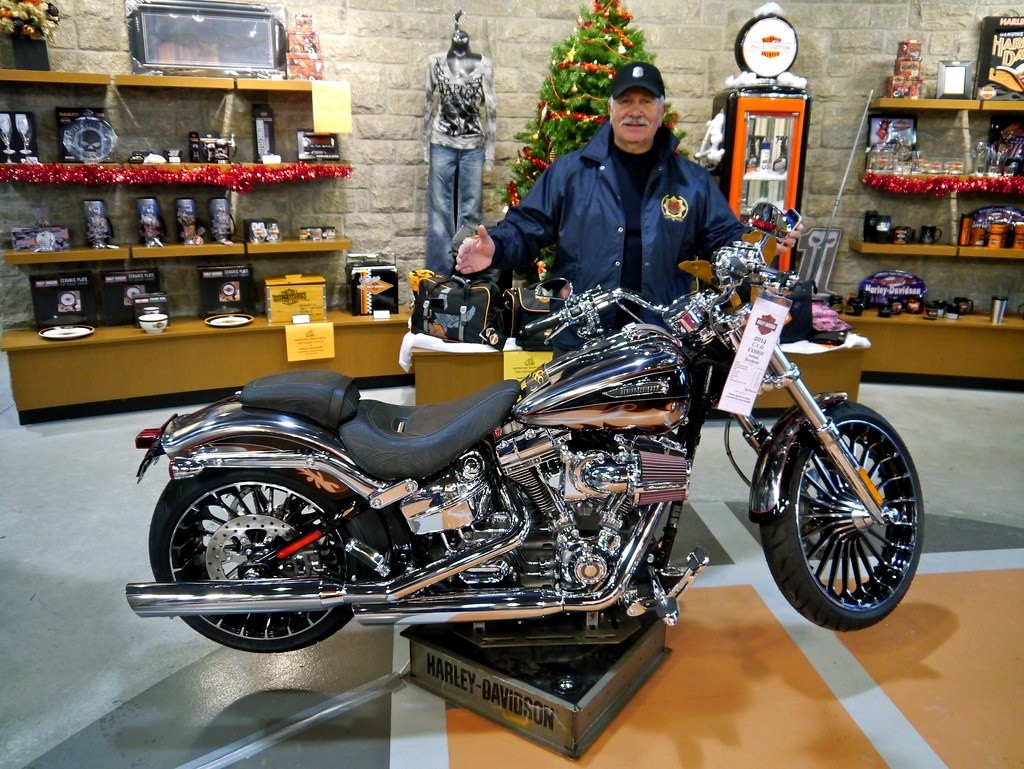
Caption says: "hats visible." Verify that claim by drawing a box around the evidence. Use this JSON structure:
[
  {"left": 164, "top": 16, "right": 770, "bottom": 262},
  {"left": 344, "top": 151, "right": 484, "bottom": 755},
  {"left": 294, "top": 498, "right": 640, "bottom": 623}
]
[{"left": 610, "top": 62, "right": 665, "bottom": 99}]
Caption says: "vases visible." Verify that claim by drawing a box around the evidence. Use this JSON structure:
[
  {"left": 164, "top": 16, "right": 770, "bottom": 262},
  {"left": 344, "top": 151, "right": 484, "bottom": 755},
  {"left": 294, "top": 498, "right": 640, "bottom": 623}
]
[{"left": 13, "top": 38, "right": 51, "bottom": 71}]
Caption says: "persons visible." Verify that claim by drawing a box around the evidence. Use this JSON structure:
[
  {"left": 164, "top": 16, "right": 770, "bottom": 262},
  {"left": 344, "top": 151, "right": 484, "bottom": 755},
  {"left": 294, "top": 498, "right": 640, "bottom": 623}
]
[
  {"left": 422, "top": 31, "right": 495, "bottom": 279},
  {"left": 456, "top": 61, "right": 804, "bottom": 382}
]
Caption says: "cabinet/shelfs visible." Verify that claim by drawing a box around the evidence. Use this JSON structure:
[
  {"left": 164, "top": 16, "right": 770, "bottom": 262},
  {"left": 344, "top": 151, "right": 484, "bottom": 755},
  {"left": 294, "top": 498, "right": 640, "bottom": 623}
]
[{"left": 0, "top": 70, "right": 1024, "bottom": 429}]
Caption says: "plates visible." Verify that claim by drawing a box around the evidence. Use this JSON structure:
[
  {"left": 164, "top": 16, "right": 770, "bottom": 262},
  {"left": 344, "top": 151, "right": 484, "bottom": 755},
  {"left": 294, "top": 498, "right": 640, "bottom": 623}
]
[
  {"left": 62, "top": 118, "right": 117, "bottom": 162},
  {"left": 38, "top": 324, "right": 94, "bottom": 341},
  {"left": 204, "top": 313, "right": 254, "bottom": 328}
]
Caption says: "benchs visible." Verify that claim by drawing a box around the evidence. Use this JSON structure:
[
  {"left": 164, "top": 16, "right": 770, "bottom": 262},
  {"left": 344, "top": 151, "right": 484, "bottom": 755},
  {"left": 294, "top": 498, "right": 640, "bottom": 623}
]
[{"left": 408, "top": 341, "right": 870, "bottom": 410}]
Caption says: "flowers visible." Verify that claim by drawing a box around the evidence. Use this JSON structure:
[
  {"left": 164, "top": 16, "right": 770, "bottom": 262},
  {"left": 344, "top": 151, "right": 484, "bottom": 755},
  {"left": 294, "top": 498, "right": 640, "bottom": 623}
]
[{"left": 0, "top": 0, "right": 60, "bottom": 39}]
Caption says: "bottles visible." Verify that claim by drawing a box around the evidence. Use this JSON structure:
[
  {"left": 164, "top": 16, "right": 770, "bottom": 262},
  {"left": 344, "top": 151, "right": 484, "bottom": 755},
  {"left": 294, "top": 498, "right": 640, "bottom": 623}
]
[
  {"left": 876, "top": 214, "right": 891, "bottom": 244},
  {"left": 862, "top": 209, "right": 878, "bottom": 243}
]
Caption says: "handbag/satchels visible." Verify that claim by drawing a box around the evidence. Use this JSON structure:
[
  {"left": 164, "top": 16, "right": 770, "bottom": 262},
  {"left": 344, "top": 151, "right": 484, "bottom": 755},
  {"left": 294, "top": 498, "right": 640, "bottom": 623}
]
[
  {"left": 741, "top": 278, "right": 818, "bottom": 344},
  {"left": 411, "top": 274, "right": 502, "bottom": 344},
  {"left": 502, "top": 278, "right": 567, "bottom": 351}
]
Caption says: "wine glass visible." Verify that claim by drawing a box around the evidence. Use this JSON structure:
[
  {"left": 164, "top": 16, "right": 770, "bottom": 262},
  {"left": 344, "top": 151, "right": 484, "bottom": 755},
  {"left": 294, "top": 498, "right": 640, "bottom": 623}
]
[
  {"left": 15, "top": 114, "right": 34, "bottom": 155},
  {"left": 0, "top": 113, "right": 16, "bottom": 155}
]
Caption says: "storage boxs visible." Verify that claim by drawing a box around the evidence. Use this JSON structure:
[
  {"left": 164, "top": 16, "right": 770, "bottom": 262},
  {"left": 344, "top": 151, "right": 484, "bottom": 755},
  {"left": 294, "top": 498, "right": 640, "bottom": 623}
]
[
  {"left": 897, "top": 41, "right": 922, "bottom": 58},
  {"left": 261, "top": 217, "right": 280, "bottom": 241},
  {"left": 345, "top": 260, "right": 399, "bottom": 315},
  {"left": 887, "top": 76, "right": 923, "bottom": 100},
  {"left": 263, "top": 275, "right": 327, "bottom": 322},
  {"left": 252, "top": 103, "right": 275, "bottom": 163},
  {"left": 286, "top": 12, "right": 324, "bottom": 80},
  {"left": 163, "top": 148, "right": 183, "bottom": 163},
  {"left": 27, "top": 269, "right": 99, "bottom": 330},
  {"left": 100, "top": 266, "right": 160, "bottom": 325},
  {"left": 894, "top": 59, "right": 921, "bottom": 75},
  {"left": 132, "top": 290, "right": 172, "bottom": 328},
  {"left": 242, "top": 219, "right": 265, "bottom": 243},
  {"left": 399, "top": 622, "right": 674, "bottom": 761},
  {"left": 296, "top": 128, "right": 340, "bottom": 161},
  {"left": 54, "top": 105, "right": 114, "bottom": 164},
  {"left": 973, "top": 15, "right": 1024, "bottom": 101},
  {"left": 0, "top": 110, "right": 40, "bottom": 164},
  {"left": 198, "top": 262, "right": 259, "bottom": 318},
  {"left": 188, "top": 131, "right": 220, "bottom": 163}
]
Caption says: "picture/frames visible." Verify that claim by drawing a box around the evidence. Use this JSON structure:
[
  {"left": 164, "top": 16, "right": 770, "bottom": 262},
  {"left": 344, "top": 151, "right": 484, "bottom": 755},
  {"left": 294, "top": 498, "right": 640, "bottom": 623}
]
[
  {"left": 125, "top": 0, "right": 290, "bottom": 79},
  {"left": 866, "top": 114, "right": 918, "bottom": 171},
  {"left": 936, "top": 61, "right": 972, "bottom": 100}
]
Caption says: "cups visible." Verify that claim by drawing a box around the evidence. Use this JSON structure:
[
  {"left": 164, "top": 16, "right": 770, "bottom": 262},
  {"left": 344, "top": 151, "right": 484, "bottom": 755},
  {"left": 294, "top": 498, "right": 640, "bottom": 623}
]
[
  {"left": 991, "top": 295, "right": 1008, "bottom": 325},
  {"left": 209, "top": 197, "right": 236, "bottom": 245},
  {"left": 175, "top": 197, "right": 200, "bottom": 245},
  {"left": 918, "top": 224, "right": 942, "bottom": 245},
  {"left": 83, "top": 199, "right": 115, "bottom": 249},
  {"left": 135, "top": 196, "right": 167, "bottom": 247}
]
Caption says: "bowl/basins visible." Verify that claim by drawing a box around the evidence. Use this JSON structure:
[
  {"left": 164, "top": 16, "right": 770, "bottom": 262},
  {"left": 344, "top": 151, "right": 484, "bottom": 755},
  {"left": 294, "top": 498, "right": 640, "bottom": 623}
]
[{"left": 138, "top": 313, "right": 168, "bottom": 334}]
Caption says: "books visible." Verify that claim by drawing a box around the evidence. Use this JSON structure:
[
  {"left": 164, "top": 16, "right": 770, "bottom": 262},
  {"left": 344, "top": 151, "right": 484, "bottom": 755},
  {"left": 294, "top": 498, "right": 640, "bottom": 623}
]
[{"left": 738, "top": 117, "right": 796, "bottom": 216}]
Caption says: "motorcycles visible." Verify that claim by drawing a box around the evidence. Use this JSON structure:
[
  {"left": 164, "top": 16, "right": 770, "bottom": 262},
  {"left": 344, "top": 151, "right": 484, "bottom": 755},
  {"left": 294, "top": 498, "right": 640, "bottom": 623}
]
[{"left": 125, "top": 208, "right": 931, "bottom": 656}]
